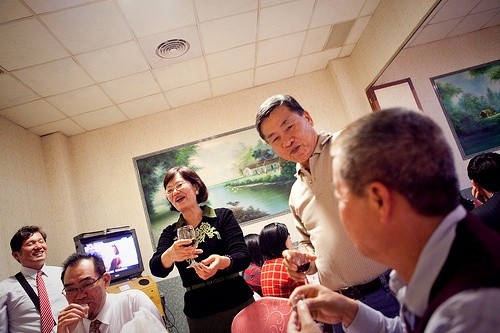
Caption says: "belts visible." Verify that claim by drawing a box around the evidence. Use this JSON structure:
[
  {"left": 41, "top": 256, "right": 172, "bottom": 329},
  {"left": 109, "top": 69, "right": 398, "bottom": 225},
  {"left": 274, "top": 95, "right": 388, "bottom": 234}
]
[{"left": 335, "top": 273, "right": 390, "bottom": 299}]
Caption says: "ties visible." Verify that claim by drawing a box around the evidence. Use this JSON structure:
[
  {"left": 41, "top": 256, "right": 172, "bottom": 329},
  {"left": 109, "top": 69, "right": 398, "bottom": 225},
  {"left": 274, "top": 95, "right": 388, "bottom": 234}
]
[
  {"left": 89, "top": 319, "right": 102, "bottom": 333},
  {"left": 36, "top": 270, "right": 53, "bottom": 333}
]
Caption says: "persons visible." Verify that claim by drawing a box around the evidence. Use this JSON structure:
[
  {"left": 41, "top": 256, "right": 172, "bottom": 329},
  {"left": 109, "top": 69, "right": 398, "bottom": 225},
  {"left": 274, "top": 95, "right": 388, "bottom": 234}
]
[
  {"left": 149, "top": 166, "right": 256, "bottom": 333},
  {"left": 287, "top": 106, "right": 500, "bottom": 333},
  {"left": 255, "top": 94, "right": 401, "bottom": 332},
  {"left": 109, "top": 242, "right": 121, "bottom": 271},
  {"left": 0, "top": 225, "right": 169, "bottom": 333},
  {"left": 467, "top": 151, "right": 500, "bottom": 222},
  {"left": 242, "top": 222, "right": 309, "bottom": 298}
]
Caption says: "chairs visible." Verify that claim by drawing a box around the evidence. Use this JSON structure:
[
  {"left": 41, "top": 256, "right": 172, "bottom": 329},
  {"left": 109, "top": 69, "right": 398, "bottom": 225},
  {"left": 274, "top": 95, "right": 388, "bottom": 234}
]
[{"left": 231, "top": 296, "right": 293, "bottom": 333}]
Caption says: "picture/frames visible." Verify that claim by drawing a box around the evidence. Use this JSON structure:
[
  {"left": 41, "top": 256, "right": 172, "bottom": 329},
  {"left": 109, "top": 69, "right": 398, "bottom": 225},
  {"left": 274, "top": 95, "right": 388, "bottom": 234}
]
[
  {"left": 430, "top": 59, "right": 500, "bottom": 160},
  {"left": 131, "top": 124, "right": 296, "bottom": 252}
]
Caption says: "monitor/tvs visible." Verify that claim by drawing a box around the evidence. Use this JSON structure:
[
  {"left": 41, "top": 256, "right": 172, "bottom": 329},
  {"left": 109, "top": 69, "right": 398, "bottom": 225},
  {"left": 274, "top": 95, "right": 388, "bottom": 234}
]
[{"left": 75, "top": 229, "right": 144, "bottom": 286}]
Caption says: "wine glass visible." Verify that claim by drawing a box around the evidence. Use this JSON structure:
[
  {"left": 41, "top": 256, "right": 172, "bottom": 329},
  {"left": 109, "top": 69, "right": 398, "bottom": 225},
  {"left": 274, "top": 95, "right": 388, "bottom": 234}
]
[
  {"left": 289, "top": 244, "right": 312, "bottom": 285},
  {"left": 177, "top": 224, "right": 202, "bottom": 269}
]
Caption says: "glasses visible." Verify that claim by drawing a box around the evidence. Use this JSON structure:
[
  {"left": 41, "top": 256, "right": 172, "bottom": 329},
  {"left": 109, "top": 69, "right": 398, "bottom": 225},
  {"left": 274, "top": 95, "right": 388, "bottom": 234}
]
[
  {"left": 61, "top": 275, "right": 102, "bottom": 297},
  {"left": 164, "top": 180, "right": 189, "bottom": 196}
]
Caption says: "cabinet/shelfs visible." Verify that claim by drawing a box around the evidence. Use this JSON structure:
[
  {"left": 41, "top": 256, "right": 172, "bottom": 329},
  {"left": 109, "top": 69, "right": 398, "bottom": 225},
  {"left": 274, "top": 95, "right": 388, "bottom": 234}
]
[{"left": 106, "top": 275, "right": 167, "bottom": 325}]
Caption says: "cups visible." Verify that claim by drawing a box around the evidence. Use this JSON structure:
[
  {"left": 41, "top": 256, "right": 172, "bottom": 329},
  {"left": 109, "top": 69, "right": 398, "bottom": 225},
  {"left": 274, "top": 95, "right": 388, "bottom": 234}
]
[
  {"left": 291, "top": 294, "right": 305, "bottom": 313},
  {"left": 81, "top": 303, "right": 89, "bottom": 318}
]
